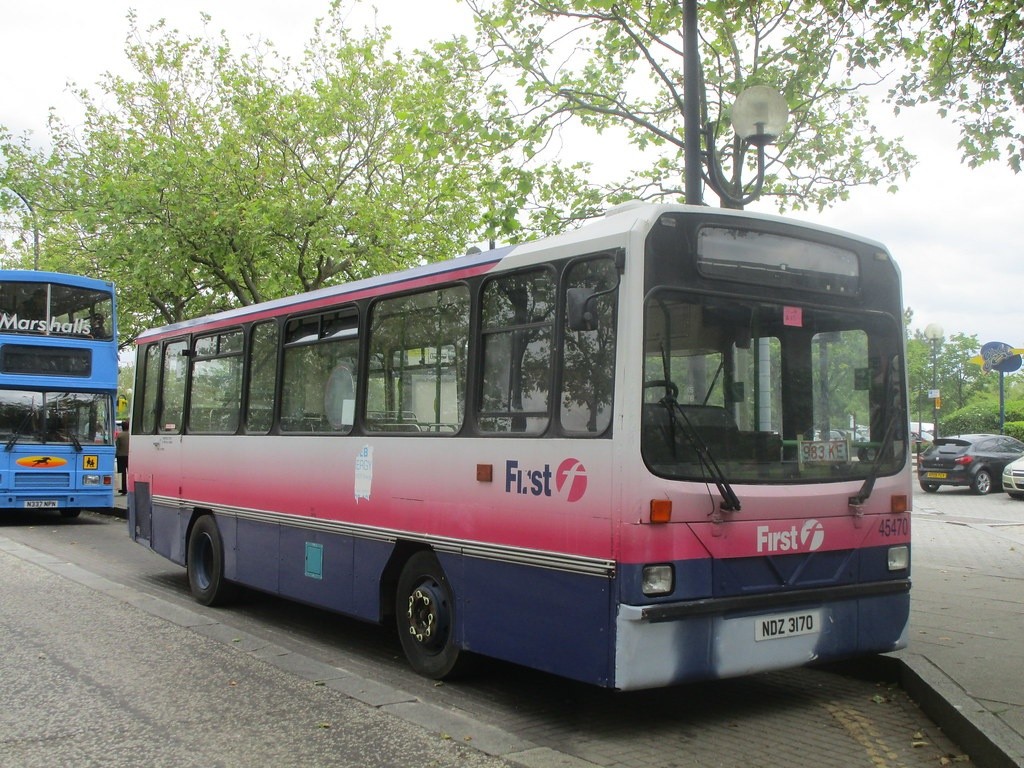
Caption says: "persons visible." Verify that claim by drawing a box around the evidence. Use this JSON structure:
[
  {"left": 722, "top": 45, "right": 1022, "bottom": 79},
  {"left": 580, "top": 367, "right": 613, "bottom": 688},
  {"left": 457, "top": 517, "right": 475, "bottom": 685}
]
[{"left": 116, "top": 419, "right": 130, "bottom": 494}]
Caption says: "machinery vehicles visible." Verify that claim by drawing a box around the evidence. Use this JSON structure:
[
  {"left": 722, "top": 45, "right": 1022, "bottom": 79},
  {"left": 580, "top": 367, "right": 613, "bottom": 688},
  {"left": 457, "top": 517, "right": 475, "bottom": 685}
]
[{"left": 0, "top": 272, "right": 128, "bottom": 519}]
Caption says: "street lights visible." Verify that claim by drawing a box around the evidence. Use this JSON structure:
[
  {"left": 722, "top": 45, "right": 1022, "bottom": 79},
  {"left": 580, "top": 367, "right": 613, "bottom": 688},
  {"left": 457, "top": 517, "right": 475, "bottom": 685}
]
[
  {"left": 707, "top": 85, "right": 789, "bottom": 433},
  {"left": 925, "top": 323, "right": 943, "bottom": 440}
]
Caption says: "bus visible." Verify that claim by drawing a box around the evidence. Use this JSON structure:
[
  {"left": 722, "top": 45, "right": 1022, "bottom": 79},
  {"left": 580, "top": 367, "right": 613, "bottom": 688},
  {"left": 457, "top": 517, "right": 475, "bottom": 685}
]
[{"left": 123, "top": 203, "right": 911, "bottom": 690}]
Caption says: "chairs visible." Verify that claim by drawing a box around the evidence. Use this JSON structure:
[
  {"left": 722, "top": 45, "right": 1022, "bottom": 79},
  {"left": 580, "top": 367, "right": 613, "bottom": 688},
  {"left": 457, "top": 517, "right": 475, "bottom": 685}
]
[{"left": 147, "top": 402, "right": 743, "bottom": 466}]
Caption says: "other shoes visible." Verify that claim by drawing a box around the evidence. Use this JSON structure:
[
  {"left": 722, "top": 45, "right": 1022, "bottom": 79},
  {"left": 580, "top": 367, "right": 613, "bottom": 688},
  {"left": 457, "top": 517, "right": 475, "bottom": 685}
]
[{"left": 118, "top": 489, "right": 128, "bottom": 496}]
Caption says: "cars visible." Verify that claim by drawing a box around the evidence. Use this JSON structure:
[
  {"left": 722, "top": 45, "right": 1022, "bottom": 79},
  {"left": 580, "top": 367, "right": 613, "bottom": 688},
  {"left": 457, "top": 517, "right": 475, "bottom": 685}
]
[
  {"left": 918, "top": 430, "right": 1024, "bottom": 495},
  {"left": 813, "top": 429, "right": 864, "bottom": 441},
  {"left": 1002, "top": 456, "right": 1024, "bottom": 497},
  {"left": 909, "top": 431, "right": 934, "bottom": 453}
]
